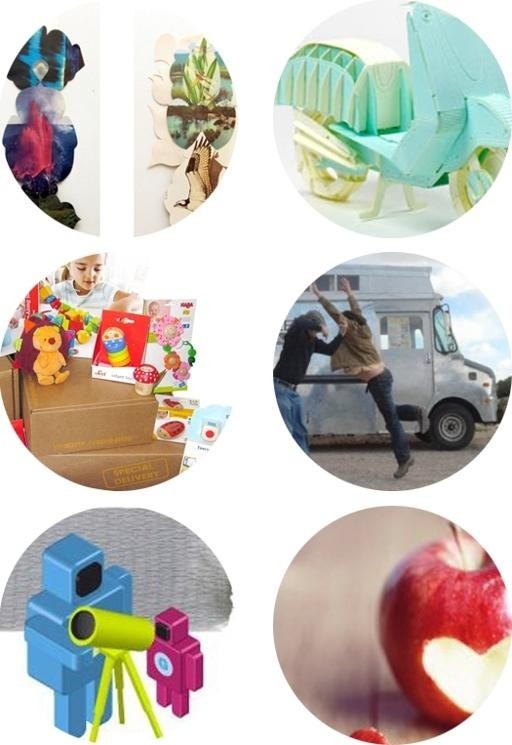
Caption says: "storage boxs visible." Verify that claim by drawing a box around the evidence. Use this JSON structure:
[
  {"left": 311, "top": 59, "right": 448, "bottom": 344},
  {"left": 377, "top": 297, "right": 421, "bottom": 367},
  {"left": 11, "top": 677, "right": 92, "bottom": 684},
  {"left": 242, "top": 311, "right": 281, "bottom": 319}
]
[{"left": 1, "top": 354, "right": 184, "bottom": 491}]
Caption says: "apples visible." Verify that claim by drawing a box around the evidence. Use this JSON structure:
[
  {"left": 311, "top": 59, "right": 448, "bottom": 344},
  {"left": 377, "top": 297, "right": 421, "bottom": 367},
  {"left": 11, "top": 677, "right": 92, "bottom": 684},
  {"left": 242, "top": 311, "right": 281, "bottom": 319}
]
[{"left": 380, "top": 524, "right": 512, "bottom": 728}]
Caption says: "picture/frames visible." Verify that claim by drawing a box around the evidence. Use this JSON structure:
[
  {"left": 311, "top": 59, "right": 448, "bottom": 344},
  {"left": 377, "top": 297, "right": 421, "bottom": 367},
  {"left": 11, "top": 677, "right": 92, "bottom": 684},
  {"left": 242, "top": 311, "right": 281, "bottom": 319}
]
[{"left": 143, "top": 32, "right": 238, "bottom": 168}]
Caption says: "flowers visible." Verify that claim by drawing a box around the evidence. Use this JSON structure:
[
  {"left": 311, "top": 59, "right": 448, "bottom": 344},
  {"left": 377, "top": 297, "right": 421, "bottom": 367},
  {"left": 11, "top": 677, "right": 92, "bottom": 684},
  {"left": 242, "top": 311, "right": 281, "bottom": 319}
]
[{"left": 182, "top": 36, "right": 222, "bottom": 111}]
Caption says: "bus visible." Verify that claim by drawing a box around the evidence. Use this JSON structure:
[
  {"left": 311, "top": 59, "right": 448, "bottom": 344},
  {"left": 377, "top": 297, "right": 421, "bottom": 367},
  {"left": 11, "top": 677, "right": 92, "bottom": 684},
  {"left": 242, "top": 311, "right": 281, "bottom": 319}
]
[{"left": 274, "top": 265, "right": 499, "bottom": 453}]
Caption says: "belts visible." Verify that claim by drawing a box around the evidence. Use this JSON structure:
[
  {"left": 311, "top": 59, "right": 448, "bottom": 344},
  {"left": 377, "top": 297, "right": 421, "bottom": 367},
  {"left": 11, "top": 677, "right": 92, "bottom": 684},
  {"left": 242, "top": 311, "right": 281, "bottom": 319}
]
[{"left": 273, "top": 378, "right": 295, "bottom": 392}]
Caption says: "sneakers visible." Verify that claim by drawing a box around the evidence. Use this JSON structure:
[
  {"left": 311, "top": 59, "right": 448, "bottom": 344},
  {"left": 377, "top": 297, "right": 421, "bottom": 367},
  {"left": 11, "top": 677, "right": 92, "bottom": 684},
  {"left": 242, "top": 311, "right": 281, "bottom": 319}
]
[{"left": 393, "top": 455, "right": 415, "bottom": 479}]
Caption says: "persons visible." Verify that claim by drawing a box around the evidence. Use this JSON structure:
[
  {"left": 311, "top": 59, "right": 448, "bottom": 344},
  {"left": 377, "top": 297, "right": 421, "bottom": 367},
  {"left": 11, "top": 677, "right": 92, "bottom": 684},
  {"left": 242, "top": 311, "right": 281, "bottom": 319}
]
[
  {"left": 309, "top": 277, "right": 429, "bottom": 479},
  {"left": 50, "top": 254, "right": 136, "bottom": 320},
  {"left": 273, "top": 309, "right": 350, "bottom": 458}
]
